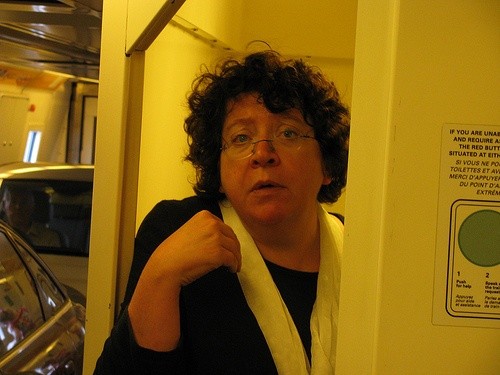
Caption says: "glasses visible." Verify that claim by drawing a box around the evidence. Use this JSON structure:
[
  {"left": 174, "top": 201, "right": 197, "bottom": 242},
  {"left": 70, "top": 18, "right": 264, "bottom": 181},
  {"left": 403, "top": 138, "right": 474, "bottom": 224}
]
[{"left": 219, "top": 123, "right": 318, "bottom": 160}]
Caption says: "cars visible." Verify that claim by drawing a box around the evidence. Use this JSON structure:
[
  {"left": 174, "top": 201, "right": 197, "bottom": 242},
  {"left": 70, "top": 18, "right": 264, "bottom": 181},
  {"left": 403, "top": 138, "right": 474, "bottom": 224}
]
[{"left": 0, "top": 222, "right": 84, "bottom": 375}]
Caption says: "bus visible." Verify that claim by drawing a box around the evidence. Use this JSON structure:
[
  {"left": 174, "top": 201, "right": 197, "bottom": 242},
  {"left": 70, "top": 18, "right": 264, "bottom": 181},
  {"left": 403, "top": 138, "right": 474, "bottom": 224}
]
[{"left": 1, "top": 165, "right": 94, "bottom": 298}]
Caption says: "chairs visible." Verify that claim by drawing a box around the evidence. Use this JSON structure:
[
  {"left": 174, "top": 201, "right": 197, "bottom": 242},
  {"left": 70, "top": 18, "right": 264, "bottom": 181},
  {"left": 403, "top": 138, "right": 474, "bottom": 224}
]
[{"left": 32, "top": 192, "right": 69, "bottom": 247}]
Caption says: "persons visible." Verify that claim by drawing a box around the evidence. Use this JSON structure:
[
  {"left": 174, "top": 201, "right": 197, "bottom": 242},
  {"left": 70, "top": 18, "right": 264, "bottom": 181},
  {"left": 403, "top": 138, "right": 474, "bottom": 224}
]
[
  {"left": 92, "top": 39, "right": 351, "bottom": 374},
  {"left": 1, "top": 185, "right": 61, "bottom": 249}
]
